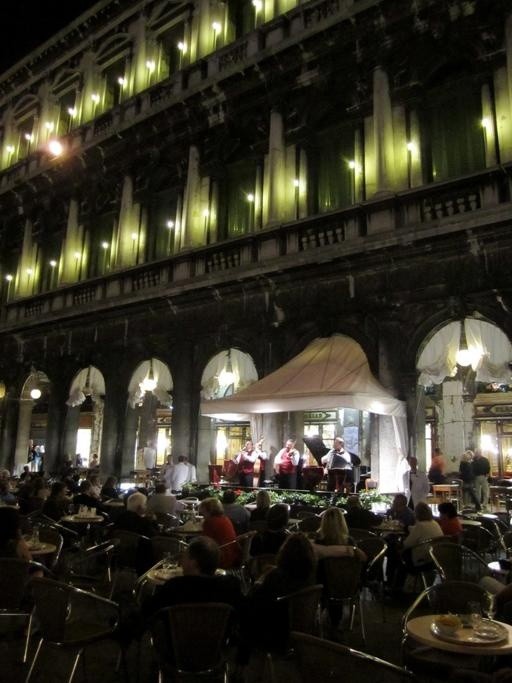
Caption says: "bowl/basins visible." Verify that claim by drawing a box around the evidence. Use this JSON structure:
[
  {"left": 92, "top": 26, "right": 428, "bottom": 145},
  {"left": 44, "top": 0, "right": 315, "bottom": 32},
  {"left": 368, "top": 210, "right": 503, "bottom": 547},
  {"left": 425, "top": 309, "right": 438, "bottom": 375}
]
[{"left": 435, "top": 618, "right": 463, "bottom": 635}]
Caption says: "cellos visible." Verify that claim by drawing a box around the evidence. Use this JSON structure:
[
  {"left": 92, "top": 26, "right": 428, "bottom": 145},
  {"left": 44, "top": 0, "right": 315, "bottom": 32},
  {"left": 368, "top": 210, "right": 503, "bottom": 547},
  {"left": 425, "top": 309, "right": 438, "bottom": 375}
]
[{"left": 252, "top": 435, "right": 265, "bottom": 487}]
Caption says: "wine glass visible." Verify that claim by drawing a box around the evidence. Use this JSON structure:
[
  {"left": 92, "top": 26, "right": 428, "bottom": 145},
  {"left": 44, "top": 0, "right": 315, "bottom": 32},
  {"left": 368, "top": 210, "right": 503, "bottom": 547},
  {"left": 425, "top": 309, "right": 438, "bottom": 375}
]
[
  {"left": 161, "top": 551, "right": 179, "bottom": 576},
  {"left": 482, "top": 593, "right": 497, "bottom": 621},
  {"left": 470, "top": 612, "right": 482, "bottom": 634}
]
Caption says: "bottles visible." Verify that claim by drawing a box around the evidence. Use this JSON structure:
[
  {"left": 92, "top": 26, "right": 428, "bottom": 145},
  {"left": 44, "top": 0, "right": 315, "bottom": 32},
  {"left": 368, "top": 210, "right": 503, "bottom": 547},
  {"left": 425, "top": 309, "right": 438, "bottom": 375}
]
[
  {"left": 31, "top": 526, "right": 39, "bottom": 548},
  {"left": 189, "top": 503, "right": 196, "bottom": 525},
  {"left": 383, "top": 515, "right": 392, "bottom": 527},
  {"left": 505, "top": 547, "right": 512, "bottom": 560}
]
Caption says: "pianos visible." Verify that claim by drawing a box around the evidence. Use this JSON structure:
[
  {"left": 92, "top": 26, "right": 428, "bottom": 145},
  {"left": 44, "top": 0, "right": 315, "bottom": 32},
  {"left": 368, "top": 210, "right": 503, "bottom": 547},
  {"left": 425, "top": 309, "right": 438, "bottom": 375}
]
[{"left": 301, "top": 437, "right": 331, "bottom": 490}]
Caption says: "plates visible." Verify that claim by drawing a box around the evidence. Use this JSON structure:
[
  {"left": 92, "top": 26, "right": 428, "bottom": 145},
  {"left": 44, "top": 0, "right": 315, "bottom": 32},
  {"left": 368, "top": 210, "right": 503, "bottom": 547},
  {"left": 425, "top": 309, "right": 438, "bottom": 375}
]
[
  {"left": 473, "top": 627, "right": 500, "bottom": 640},
  {"left": 457, "top": 618, "right": 481, "bottom": 628}
]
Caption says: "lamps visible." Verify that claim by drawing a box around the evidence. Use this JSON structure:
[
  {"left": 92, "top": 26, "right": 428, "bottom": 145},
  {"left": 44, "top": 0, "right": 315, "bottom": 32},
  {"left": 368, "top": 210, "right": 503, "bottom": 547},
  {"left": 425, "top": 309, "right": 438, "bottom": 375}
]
[
  {"left": 223, "top": 350, "right": 236, "bottom": 387},
  {"left": 31, "top": 372, "right": 42, "bottom": 399},
  {"left": 455, "top": 319, "right": 471, "bottom": 367},
  {"left": 141, "top": 356, "right": 157, "bottom": 392},
  {"left": 82, "top": 367, "right": 94, "bottom": 399}
]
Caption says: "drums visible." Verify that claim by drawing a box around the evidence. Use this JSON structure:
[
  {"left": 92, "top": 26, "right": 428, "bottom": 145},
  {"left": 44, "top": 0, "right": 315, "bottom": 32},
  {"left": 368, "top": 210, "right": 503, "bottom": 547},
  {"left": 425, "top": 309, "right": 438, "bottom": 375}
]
[{"left": 326, "top": 469, "right": 355, "bottom": 493}]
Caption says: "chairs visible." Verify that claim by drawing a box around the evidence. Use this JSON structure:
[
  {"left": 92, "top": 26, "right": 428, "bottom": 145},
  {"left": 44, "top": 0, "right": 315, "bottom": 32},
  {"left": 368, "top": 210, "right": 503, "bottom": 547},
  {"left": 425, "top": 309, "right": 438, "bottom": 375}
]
[{"left": 1, "top": 473, "right": 511, "bottom": 681}]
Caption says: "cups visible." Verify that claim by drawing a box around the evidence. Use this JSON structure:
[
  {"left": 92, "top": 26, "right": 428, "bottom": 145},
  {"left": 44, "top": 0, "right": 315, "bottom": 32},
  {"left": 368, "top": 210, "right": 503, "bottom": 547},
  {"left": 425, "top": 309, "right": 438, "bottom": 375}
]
[
  {"left": 68, "top": 502, "right": 97, "bottom": 520},
  {"left": 195, "top": 515, "right": 204, "bottom": 524}
]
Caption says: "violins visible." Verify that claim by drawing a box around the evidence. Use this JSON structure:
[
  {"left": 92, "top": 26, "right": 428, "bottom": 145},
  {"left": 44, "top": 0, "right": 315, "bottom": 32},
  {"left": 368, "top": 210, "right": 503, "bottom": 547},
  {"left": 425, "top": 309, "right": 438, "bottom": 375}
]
[{"left": 286, "top": 450, "right": 295, "bottom": 459}]
